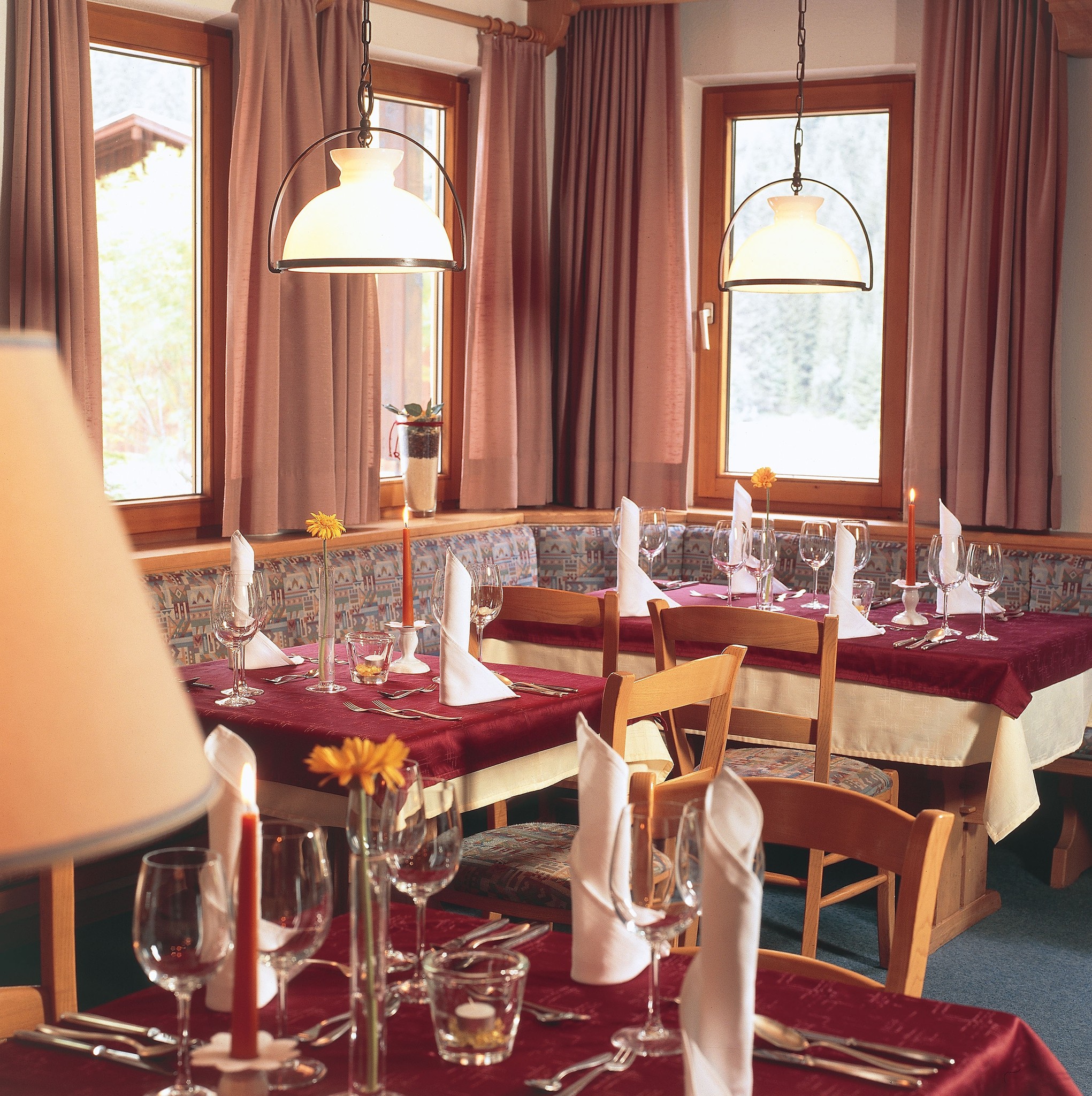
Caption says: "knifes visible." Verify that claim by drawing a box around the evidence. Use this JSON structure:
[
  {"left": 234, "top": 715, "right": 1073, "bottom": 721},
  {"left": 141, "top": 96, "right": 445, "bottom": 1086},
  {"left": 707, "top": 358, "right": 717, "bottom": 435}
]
[
  {"left": 789, "top": 1027, "right": 951, "bottom": 1068},
  {"left": 13, "top": 1028, "right": 177, "bottom": 1076},
  {"left": 753, "top": 1047, "right": 922, "bottom": 1090},
  {"left": 407, "top": 918, "right": 509, "bottom": 964},
  {"left": 59, "top": 1011, "right": 209, "bottom": 1049},
  {"left": 661, "top": 581, "right": 699, "bottom": 591}
]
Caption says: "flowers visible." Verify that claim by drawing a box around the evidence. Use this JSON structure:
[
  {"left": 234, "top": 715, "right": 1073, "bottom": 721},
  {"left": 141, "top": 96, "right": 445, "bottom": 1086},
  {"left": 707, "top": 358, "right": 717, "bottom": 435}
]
[
  {"left": 751, "top": 467, "right": 778, "bottom": 603},
  {"left": 382, "top": 398, "right": 444, "bottom": 432},
  {"left": 305, "top": 511, "right": 346, "bottom": 682},
  {"left": 303, "top": 733, "right": 410, "bottom": 1090}
]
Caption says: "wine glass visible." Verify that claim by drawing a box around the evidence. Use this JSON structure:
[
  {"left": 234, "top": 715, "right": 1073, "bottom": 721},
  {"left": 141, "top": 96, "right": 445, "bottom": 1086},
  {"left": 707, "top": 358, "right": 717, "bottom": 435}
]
[
  {"left": 965, "top": 542, "right": 1004, "bottom": 641},
  {"left": 430, "top": 563, "right": 503, "bottom": 667},
  {"left": 210, "top": 569, "right": 264, "bottom": 708},
  {"left": 928, "top": 535, "right": 968, "bottom": 635},
  {"left": 132, "top": 759, "right": 767, "bottom": 1096},
  {"left": 610, "top": 505, "right": 871, "bottom": 612}
]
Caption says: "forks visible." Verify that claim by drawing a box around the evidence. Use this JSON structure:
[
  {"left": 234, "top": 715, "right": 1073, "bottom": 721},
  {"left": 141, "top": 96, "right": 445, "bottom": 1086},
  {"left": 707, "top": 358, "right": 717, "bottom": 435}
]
[
  {"left": 177, "top": 651, "right": 579, "bottom": 719},
  {"left": 553, "top": 1044, "right": 639, "bottom": 1096},
  {"left": 287, "top": 1010, "right": 350, "bottom": 1042},
  {"left": 287, "top": 655, "right": 318, "bottom": 663},
  {"left": 773, "top": 589, "right": 1024, "bottom": 650}
]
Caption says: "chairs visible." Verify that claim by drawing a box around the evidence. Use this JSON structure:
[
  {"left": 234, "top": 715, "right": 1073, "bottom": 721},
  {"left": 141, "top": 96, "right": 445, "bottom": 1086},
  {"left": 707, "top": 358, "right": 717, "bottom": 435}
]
[{"left": 1, "top": 584, "right": 1091, "bottom": 1040}]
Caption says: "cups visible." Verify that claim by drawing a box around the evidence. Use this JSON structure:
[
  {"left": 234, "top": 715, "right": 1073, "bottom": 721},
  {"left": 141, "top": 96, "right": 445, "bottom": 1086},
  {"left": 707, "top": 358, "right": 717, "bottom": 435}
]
[
  {"left": 852, "top": 579, "right": 876, "bottom": 620},
  {"left": 344, "top": 631, "right": 396, "bottom": 685},
  {"left": 420, "top": 945, "right": 530, "bottom": 1067}
]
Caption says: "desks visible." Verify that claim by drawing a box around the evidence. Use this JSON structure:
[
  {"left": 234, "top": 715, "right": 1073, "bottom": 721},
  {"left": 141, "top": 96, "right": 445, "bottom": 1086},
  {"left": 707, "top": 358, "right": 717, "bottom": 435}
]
[
  {"left": 0, "top": 901, "right": 1084, "bottom": 1095},
  {"left": 179, "top": 638, "right": 665, "bottom": 912},
  {"left": 470, "top": 579, "right": 1091, "bottom": 957}
]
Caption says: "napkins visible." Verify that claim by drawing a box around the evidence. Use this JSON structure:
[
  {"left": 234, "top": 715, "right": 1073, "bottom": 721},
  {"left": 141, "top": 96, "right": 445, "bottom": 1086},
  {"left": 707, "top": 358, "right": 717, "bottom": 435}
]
[
  {"left": 440, "top": 546, "right": 521, "bottom": 706},
  {"left": 201, "top": 712, "right": 764, "bottom": 1096},
  {"left": 230, "top": 530, "right": 305, "bottom": 670},
  {"left": 616, "top": 480, "right": 1006, "bottom": 639}
]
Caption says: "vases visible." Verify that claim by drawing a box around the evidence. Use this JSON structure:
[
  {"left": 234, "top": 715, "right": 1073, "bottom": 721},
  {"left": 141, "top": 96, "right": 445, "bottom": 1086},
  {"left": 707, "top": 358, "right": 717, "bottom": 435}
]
[
  {"left": 306, "top": 568, "right": 348, "bottom": 694},
  {"left": 396, "top": 416, "right": 441, "bottom": 519},
  {"left": 748, "top": 519, "right": 785, "bottom": 612},
  {"left": 350, "top": 855, "right": 390, "bottom": 1096}
]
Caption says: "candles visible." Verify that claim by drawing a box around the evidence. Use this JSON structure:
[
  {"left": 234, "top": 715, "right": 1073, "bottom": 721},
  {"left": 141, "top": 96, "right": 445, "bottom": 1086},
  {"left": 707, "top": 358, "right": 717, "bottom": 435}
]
[
  {"left": 905, "top": 488, "right": 916, "bottom": 586},
  {"left": 402, "top": 522, "right": 414, "bottom": 626},
  {"left": 229, "top": 762, "right": 261, "bottom": 1061}
]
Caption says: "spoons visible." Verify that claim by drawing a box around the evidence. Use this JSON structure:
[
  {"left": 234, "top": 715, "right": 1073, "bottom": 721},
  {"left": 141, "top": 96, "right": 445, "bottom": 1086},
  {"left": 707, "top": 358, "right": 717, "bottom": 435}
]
[
  {"left": 310, "top": 991, "right": 401, "bottom": 1047},
  {"left": 523, "top": 1051, "right": 613, "bottom": 1092},
  {"left": 653, "top": 580, "right": 683, "bottom": 590},
  {"left": 36, "top": 1022, "right": 198, "bottom": 1060},
  {"left": 754, "top": 1013, "right": 938, "bottom": 1076}
]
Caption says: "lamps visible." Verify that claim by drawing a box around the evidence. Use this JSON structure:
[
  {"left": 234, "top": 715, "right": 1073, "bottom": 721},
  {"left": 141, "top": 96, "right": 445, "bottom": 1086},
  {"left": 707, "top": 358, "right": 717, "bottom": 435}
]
[
  {"left": 717, "top": 0, "right": 873, "bottom": 294},
  {"left": 267, "top": 0, "right": 467, "bottom": 274},
  {"left": 0, "top": 328, "right": 225, "bottom": 882}
]
